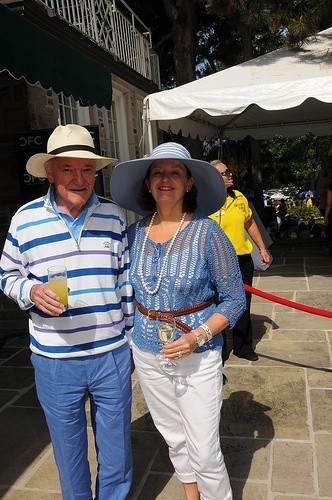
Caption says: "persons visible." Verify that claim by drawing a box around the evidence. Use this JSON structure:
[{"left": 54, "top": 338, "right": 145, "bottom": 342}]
[
  {"left": 263, "top": 194, "right": 314, "bottom": 244},
  {"left": 207, "top": 160, "right": 271, "bottom": 366},
  {"left": 324, "top": 182, "right": 332, "bottom": 257},
  {"left": 120, "top": 143, "right": 247, "bottom": 500},
  {"left": 0, "top": 124, "right": 138, "bottom": 500}
]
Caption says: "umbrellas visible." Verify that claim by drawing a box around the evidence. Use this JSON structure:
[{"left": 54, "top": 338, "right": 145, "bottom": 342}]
[
  {"left": 270, "top": 192, "right": 290, "bottom": 200},
  {"left": 299, "top": 191, "right": 320, "bottom": 198}
]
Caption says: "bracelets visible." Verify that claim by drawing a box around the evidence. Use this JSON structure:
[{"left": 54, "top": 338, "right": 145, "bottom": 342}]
[
  {"left": 199, "top": 324, "right": 213, "bottom": 342},
  {"left": 259, "top": 250, "right": 269, "bottom": 253}
]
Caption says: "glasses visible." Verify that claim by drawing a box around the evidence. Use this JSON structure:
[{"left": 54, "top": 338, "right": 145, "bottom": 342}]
[{"left": 221, "top": 170, "right": 231, "bottom": 176}]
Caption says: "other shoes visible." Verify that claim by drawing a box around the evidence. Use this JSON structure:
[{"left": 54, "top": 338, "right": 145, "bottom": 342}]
[
  {"left": 234, "top": 352, "right": 258, "bottom": 361},
  {"left": 222, "top": 373, "right": 228, "bottom": 385}
]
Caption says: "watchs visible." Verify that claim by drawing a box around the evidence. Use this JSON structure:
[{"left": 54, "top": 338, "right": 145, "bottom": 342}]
[{"left": 190, "top": 329, "right": 206, "bottom": 347}]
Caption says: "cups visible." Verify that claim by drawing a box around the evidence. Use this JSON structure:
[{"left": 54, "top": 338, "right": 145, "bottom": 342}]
[{"left": 47, "top": 265, "right": 72, "bottom": 313}]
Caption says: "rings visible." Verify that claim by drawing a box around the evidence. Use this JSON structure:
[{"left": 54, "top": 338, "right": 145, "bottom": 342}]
[{"left": 177, "top": 350, "right": 182, "bottom": 358}]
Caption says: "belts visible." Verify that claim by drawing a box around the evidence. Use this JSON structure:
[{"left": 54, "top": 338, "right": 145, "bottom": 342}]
[{"left": 133, "top": 301, "right": 211, "bottom": 334}]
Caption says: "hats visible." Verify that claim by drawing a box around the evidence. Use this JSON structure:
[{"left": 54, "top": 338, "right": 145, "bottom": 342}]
[
  {"left": 26, "top": 125, "right": 118, "bottom": 178},
  {"left": 110, "top": 143, "right": 226, "bottom": 216}
]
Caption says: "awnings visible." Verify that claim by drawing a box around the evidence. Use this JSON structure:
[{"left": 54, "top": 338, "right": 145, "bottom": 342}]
[{"left": 0, "top": 2, "right": 113, "bottom": 114}]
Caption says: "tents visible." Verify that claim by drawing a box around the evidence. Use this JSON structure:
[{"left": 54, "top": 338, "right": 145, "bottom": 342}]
[{"left": 142, "top": 25, "right": 332, "bottom": 156}]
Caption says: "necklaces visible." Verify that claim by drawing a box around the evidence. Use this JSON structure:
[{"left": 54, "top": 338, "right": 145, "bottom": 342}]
[{"left": 139, "top": 210, "right": 187, "bottom": 295}]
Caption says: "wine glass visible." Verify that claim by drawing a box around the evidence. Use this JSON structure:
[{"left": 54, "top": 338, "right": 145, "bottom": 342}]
[{"left": 156, "top": 313, "right": 180, "bottom": 371}]
